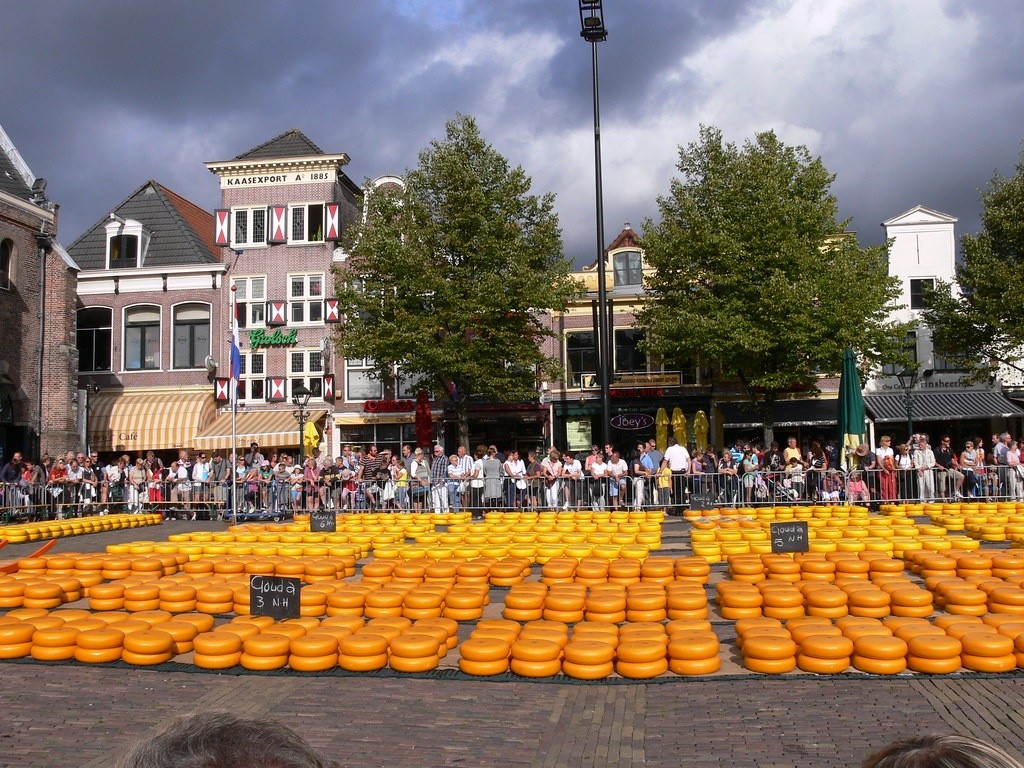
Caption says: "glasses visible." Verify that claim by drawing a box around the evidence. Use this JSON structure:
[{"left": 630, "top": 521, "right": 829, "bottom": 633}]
[
  {"left": 434, "top": 450, "right": 438, "bottom": 452},
  {"left": 79, "top": 456, "right": 83, "bottom": 458},
  {"left": 202, "top": 456, "right": 206, "bottom": 459},
  {"left": 966, "top": 446, "right": 970, "bottom": 448},
  {"left": 651, "top": 446, "right": 656, "bottom": 447},
  {"left": 592, "top": 450, "right": 597, "bottom": 451},
  {"left": 240, "top": 459, "right": 245, "bottom": 461}
]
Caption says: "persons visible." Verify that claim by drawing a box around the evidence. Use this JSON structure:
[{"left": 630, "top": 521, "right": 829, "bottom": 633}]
[
  {"left": 0, "top": 438, "right": 1024, "bottom": 526},
  {"left": 862, "top": 730, "right": 1023, "bottom": 768},
  {"left": 116, "top": 708, "right": 337, "bottom": 768}
]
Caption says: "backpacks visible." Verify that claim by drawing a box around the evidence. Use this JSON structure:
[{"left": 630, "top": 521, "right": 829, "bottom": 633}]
[
  {"left": 757, "top": 480, "right": 768, "bottom": 498},
  {"left": 414, "top": 460, "right": 429, "bottom": 480}
]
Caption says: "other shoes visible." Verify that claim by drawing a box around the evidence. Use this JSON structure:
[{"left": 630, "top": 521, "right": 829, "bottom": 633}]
[
  {"left": 794, "top": 498, "right": 801, "bottom": 500},
  {"left": 0, "top": 491, "right": 1024, "bottom": 526}
]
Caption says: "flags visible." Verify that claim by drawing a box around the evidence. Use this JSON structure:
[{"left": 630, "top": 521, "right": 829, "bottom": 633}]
[{"left": 228, "top": 301, "right": 240, "bottom": 406}]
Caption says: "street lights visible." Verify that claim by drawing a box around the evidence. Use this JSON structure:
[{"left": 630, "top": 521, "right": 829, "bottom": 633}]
[
  {"left": 31, "top": 229, "right": 53, "bottom": 467},
  {"left": 896, "top": 367, "right": 919, "bottom": 445},
  {"left": 577, "top": 0, "right": 613, "bottom": 460},
  {"left": 290, "top": 380, "right": 312, "bottom": 509}
]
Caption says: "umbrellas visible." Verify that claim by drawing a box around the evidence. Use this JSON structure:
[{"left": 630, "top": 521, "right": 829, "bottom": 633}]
[
  {"left": 840, "top": 345, "right": 866, "bottom": 473},
  {"left": 415, "top": 387, "right": 432, "bottom": 448},
  {"left": 656, "top": 406, "right": 709, "bottom": 453}
]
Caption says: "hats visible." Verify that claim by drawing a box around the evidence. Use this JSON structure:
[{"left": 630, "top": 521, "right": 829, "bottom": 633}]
[
  {"left": 294, "top": 464, "right": 303, "bottom": 470},
  {"left": 341, "top": 468, "right": 351, "bottom": 480},
  {"left": 261, "top": 460, "right": 270, "bottom": 466},
  {"left": 857, "top": 443, "right": 868, "bottom": 456}
]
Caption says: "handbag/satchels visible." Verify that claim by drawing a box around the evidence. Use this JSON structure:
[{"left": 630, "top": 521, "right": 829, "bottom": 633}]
[
  {"left": 383, "top": 478, "right": 394, "bottom": 501},
  {"left": 214, "top": 478, "right": 219, "bottom": 486},
  {"left": 883, "top": 456, "right": 892, "bottom": 473},
  {"left": 592, "top": 478, "right": 603, "bottom": 497},
  {"left": 457, "top": 478, "right": 465, "bottom": 493},
  {"left": 137, "top": 482, "right": 145, "bottom": 493},
  {"left": 539, "top": 471, "right": 557, "bottom": 489}
]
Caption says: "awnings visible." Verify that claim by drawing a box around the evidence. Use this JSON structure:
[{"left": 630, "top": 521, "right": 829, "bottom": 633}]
[{"left": 862, "top": 389, "right": 1024, "bottom": 423}]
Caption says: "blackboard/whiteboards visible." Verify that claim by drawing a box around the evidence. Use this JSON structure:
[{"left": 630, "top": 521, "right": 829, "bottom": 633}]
[
  {"left": 248, "top": 573, "right": 301, "bottom": 617},
  {"left": 309, "top": 510, "right": 336, "bottom": 532},
  {"left": 769, "top": 520, "right": 809, "bottom": 555}
]
